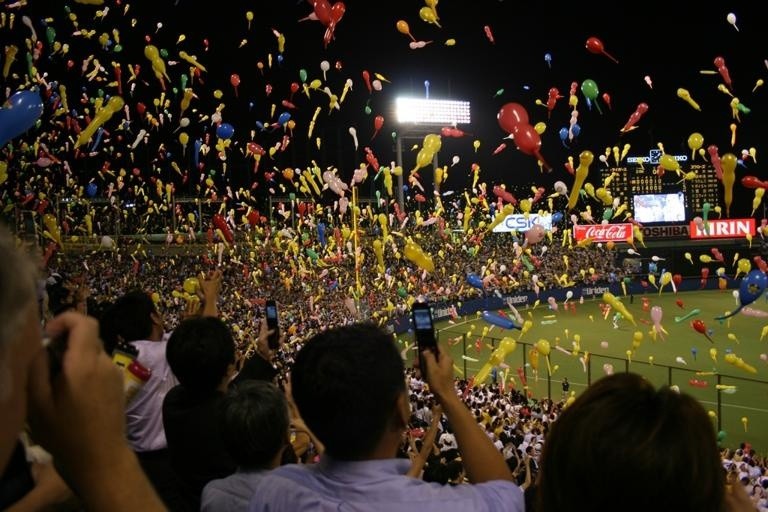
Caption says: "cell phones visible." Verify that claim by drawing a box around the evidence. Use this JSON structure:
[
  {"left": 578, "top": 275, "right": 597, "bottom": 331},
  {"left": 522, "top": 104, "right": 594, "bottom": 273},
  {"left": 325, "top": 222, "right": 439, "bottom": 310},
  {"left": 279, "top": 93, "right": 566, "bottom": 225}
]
[
  {"left": 413, "top": 302, "right": 439, "bottom": 381},
  {"left": 266, "top": 299, "right": 279, "bottom": 349}
]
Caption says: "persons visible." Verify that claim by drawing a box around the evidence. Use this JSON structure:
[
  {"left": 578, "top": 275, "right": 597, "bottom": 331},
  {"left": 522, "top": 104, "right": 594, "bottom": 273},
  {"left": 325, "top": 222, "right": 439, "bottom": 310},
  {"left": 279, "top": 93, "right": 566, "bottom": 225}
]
[{"left": 0, "top": 229, "right": 768, "bottom": 512}]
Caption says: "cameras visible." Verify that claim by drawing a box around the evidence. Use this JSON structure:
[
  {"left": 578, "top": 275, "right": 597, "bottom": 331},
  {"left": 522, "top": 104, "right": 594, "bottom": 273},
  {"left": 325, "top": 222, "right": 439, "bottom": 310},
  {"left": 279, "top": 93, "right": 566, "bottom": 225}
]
[{"left": 39, "top": 331, "right": 70, "bottom": 389}]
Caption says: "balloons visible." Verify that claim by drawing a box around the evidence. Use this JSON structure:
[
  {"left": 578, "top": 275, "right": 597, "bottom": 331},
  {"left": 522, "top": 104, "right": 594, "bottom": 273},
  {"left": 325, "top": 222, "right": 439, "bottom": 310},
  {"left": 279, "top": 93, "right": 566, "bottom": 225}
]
[{"left": 1, "top": 1, "right": 768, "bottom": 439}]
